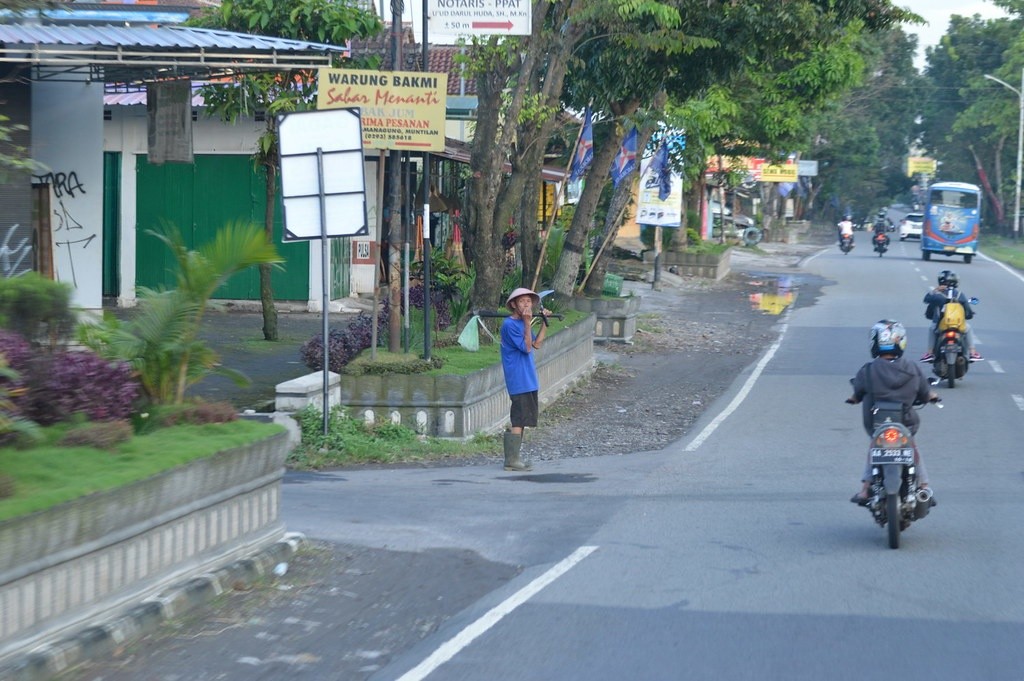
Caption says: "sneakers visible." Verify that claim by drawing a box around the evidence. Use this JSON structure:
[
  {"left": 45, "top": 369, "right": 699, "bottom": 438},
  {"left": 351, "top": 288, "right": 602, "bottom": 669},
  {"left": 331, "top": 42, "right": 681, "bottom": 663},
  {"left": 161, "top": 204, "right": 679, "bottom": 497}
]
[
  {"left": 968, "top": 352, "right": 984, "bottom": 361},
  {"left": 920, "top": 353, "right": 935, "bottom": 363}
]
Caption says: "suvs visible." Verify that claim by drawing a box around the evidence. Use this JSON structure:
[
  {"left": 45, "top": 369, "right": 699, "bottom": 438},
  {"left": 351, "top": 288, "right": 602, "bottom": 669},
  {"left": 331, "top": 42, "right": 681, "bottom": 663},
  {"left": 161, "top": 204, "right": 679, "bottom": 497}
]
[{"left": 899, "top": 213, "right": 924, "bottom": 241}]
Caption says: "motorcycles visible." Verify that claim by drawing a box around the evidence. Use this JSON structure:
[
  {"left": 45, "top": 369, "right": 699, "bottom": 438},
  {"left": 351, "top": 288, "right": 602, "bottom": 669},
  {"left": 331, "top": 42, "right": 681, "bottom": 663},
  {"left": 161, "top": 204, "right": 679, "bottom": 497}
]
[
  {"left": 845, "top": 376, "right": 941, "bottom": 549},
  {"left": 872, "top": 225, "right": 894, "bottom": 257},
  {"left": 932, "top": 296, "right": 979, "bottom": 387},
  {"left": 840, "top": 234, "right": 852, "bottom": 255}
]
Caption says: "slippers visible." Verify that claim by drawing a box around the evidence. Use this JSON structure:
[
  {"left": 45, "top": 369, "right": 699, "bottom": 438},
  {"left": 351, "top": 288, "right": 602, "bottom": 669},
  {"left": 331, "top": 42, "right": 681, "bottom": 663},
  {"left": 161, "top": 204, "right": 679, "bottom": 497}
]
[
  {"left": 851, "top": 492, "right": 868, "bottom": 504},
  {"left": 920, "top": 486, "right": 936, "bottom": 506}
]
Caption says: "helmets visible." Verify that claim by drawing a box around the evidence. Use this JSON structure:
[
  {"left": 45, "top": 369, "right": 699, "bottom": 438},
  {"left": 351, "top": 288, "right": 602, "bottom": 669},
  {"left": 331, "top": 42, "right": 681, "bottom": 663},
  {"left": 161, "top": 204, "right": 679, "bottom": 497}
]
[
  {"left": 870, "top": 318, "right": 907, "bottom": 357},
  {"left": 877, "top": 209, "right": 887, "bottom": 215},
  {"left": 938, "top": 270, "right": 958, "bottom": 288}
]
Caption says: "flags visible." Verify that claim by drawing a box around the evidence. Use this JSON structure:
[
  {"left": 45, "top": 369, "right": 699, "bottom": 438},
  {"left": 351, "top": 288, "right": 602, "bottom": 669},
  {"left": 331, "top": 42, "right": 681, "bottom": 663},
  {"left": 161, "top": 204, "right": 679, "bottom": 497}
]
[
  {"left": 609, "top": 125, "right": 637, "bottom": 190},
  {"left": 569, "top": 106, "right": 593, "bottom": 181},
  {"left": 649, "top": 138, "right": 671, "bottom": 202}
]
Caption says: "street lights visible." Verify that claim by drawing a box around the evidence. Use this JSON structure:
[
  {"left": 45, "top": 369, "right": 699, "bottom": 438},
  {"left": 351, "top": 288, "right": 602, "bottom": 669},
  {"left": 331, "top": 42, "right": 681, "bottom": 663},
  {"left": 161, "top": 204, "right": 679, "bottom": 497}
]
[{"left": 984, "top": 74, "right": 1024, "bottom": 233}]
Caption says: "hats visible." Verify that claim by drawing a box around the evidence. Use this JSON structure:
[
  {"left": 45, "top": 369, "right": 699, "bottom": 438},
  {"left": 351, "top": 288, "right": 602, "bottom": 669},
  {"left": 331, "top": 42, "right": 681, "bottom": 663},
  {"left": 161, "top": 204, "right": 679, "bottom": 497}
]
[{"left": 507, "top": 288, "right": 539, "bottom": 310}]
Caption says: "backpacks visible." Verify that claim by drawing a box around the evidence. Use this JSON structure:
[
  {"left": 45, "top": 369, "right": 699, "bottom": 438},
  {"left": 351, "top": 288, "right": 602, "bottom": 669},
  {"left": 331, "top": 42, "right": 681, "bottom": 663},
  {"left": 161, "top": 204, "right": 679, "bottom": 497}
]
[{"left": 936, "top": 290, "right": 966, "bottom": 333}]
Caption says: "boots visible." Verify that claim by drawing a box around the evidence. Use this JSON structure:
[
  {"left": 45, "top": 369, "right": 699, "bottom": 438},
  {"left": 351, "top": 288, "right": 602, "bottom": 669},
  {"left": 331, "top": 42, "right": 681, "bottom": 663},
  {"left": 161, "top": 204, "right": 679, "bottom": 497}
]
[{"left": 504, "top": 430, "right": 530, "bottom": 471}]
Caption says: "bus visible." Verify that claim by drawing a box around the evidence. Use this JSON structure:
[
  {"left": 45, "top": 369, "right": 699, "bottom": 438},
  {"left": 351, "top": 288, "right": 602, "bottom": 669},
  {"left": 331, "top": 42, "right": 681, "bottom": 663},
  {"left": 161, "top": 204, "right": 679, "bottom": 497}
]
[{"left": 922, "top": 181, "right": 983, "bottom": 263}]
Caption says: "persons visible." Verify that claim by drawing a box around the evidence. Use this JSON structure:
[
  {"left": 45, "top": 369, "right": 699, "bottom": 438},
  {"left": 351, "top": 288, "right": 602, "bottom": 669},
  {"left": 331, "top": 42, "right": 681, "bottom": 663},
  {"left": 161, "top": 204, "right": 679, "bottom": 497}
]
[
  {"left": 919, "top": 270, "right": 984, "bottom": 363},
  {"left": 500, "top": 288, "right": 551, "bottom": 471},
  {"left": 849, "top": 319, "right": 939, "bottom": 503},
  {"left": 871, "top": 211, "right": 891, "bottom": 249},
  {"left": 835, "top": 214, "right": 856, "bottom": 247}
]
